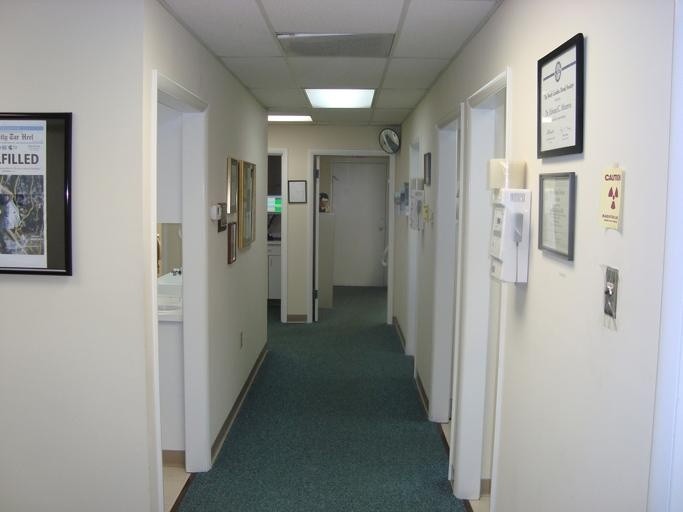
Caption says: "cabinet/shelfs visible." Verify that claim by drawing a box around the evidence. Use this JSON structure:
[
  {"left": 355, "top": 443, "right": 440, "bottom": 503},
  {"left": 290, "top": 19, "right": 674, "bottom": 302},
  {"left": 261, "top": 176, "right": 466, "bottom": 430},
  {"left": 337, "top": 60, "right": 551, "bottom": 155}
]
[{"left": 266, "top": 245, "right": 281, "bottom": 299}]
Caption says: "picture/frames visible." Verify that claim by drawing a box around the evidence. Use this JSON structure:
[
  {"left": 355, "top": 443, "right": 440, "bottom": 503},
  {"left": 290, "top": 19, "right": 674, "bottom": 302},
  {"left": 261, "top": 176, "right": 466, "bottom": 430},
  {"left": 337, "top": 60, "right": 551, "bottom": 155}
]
[
  {"left": 288, "top": 180, "right": 307, "bottom": 204},
  {"left": 227, "top": 155, "right": 256, "bottom": 264},
  {"left": 423, "top": 153, "right": 430, "bottom": 186},
  {"left": 536, "top": 31, "right": 585, "bottom": 261},
  {"left": 0, "top": 111, "right": 72, "bottom": 277}
]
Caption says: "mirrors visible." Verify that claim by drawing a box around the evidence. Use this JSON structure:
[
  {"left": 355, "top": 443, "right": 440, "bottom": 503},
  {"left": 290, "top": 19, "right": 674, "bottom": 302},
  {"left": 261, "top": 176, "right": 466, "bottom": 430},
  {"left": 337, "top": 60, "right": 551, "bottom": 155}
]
[{"left": 157, "top": 224, "right": 182, "bottom": 296}]
